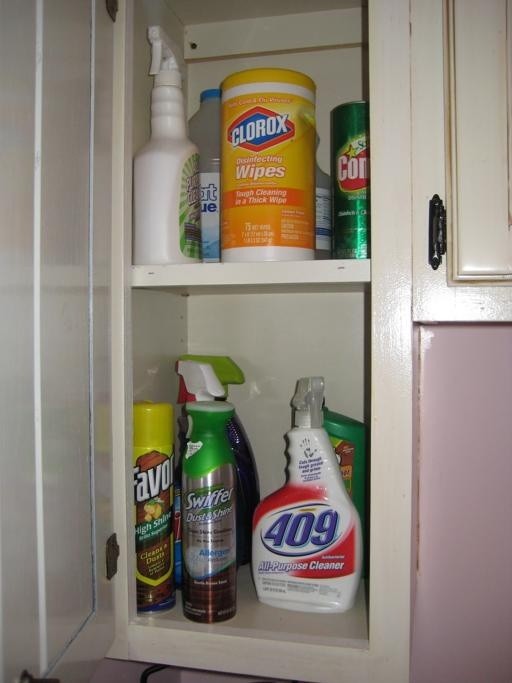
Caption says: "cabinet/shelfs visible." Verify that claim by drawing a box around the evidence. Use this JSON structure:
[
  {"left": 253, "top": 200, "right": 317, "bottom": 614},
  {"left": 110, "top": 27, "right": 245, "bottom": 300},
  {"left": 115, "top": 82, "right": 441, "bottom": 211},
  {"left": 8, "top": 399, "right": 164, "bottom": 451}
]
[{"left": 0, "top": 1, "right": 509, "bottom": 683}]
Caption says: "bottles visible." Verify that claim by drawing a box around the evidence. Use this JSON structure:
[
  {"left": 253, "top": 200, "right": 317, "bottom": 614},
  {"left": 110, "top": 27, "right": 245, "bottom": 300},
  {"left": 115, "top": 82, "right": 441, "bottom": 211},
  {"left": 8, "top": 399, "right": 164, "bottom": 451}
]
[
  {"left": 133, "top": 400, "right": 174, "bottom": 614},
  {"left": 189, "top": 69, "right": 333, "bottom": 262}
]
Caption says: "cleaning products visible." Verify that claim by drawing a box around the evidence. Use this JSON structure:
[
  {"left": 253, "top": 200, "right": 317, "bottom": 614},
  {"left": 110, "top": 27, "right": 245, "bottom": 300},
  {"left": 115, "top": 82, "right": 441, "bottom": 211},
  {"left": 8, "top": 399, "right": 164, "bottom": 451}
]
[
  {"left": 135, "top": 22, "right": 200, "bottom": 264},
  {"left": 250, "top": 379, "right": 363, "bottom": 613},
  {"left": 172, "top": 352, "right": 244, "bottom": 623}
]
